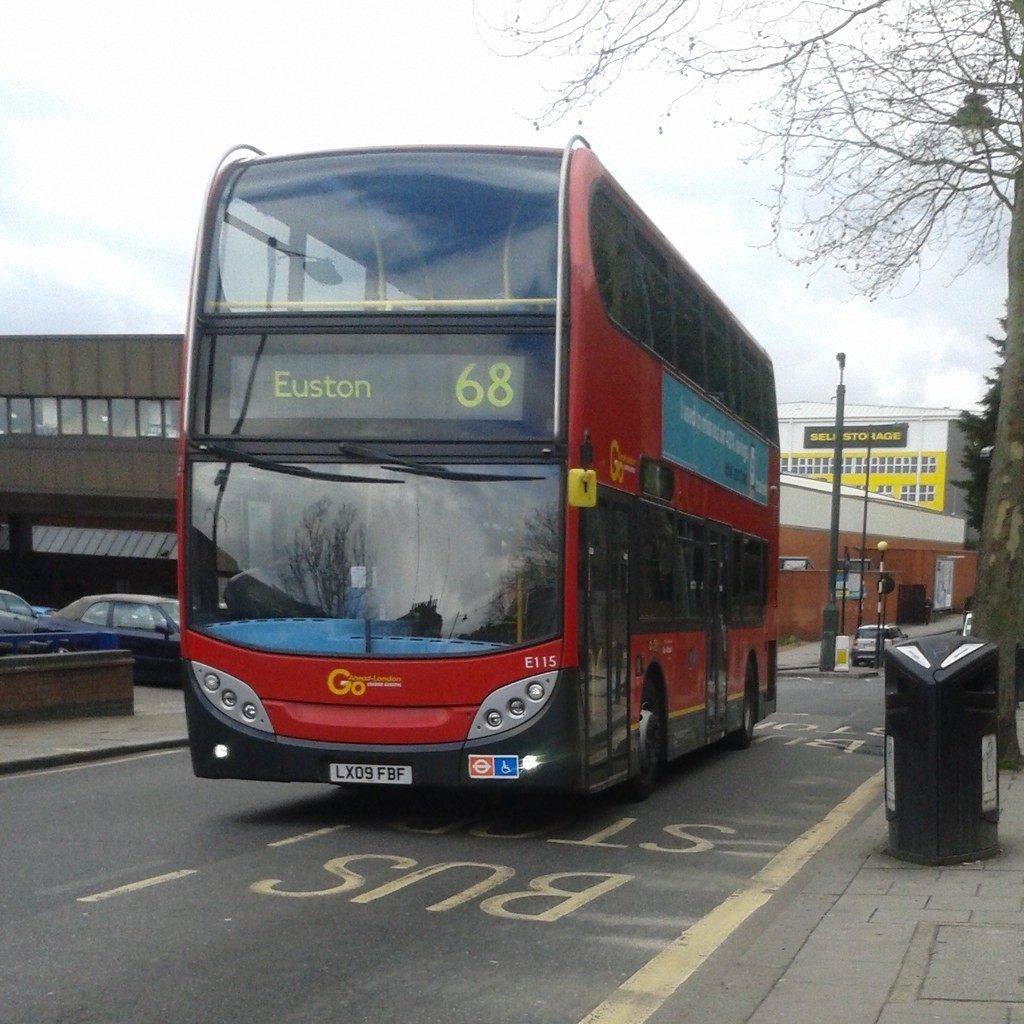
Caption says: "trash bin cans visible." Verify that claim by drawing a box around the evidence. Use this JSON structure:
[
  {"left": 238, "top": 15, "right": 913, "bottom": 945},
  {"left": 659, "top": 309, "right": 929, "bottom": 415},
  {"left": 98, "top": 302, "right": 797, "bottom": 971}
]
[{"left": 885, "top": 633, "right": 1000, "bottom": 867}]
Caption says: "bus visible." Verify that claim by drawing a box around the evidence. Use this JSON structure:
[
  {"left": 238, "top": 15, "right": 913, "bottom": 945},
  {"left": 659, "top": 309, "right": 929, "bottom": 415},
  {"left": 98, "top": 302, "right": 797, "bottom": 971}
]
[{"left": 174, "top": 133, "right": 780, "bottom": 801}]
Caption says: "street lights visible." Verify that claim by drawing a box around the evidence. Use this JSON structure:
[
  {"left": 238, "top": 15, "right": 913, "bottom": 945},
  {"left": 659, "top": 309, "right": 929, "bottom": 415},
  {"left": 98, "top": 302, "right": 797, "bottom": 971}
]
[
  {"left": 817, "top": 353, "right": 846, "bottom": 672},
  {"left": 950, "top": 81, "right": 1003, "bottom": 135}
]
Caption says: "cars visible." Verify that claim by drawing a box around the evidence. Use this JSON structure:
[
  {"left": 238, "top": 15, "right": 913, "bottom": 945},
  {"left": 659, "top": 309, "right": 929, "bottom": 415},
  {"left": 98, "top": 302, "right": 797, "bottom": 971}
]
[
  {"left": 851, "top": 623, "right": 908, "bottom": 666},
  {"left": 50, "top": 594, "right": 182, "bottom": 688},
  {"left": 0, "top": 590, "right": 60, "bottom": 634}
]
